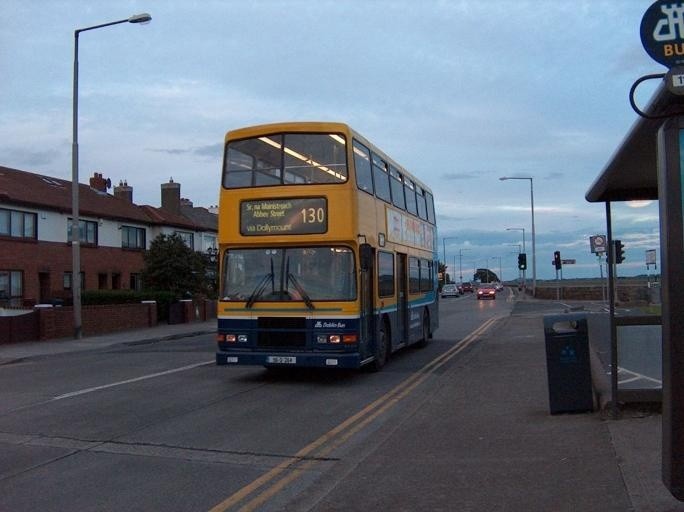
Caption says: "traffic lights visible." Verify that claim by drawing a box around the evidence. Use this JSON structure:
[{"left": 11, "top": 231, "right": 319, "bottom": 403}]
[
  {"left": 554, "top": 250, "right": 562, "bottom": 271},
  {"left": 605, "top": 240, "right": 610, "bottom": 266},
  {"left": 615, "top": 240, "right": 625, "bottom": 264}
]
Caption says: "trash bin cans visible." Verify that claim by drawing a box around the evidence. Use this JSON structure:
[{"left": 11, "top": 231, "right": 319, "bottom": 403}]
[
  {"left": 543, "top": 316, "right": 593, "bottom": 415},
  {"left": 650, "top": 283, "right": 660, "bottom": 304}
]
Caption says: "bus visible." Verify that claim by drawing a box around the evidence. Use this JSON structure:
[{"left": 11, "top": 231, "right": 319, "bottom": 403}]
[{"left": 212, "top": 115, "right": 445, "bottom": 379}]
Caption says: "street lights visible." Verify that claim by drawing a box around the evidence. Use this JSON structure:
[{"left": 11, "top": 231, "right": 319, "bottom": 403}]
[
  {"left": 459, "top": 248, "right": 471, "bottom": 282},
  {"left": 453, "top": 254, "right": 464, "bottom": 283},
  {"left": 491, "top": 255, "right": 503, "bottom": 286},
  {"left": 443, "top": 236, "right": 459, "bottom": 285},
  {"left": 507, "top": 228, "right": 527, "bottom": 299},
  {"left": 498, "top": 173, "right": 537, "bottom": 296},
  {"left": 69, "top": 10, "right": 159, "bottom": 339}
]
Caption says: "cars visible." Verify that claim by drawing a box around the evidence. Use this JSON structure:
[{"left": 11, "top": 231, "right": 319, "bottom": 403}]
[{"left": 442, "top": 281, "right": 506, "bottom": 300}]
